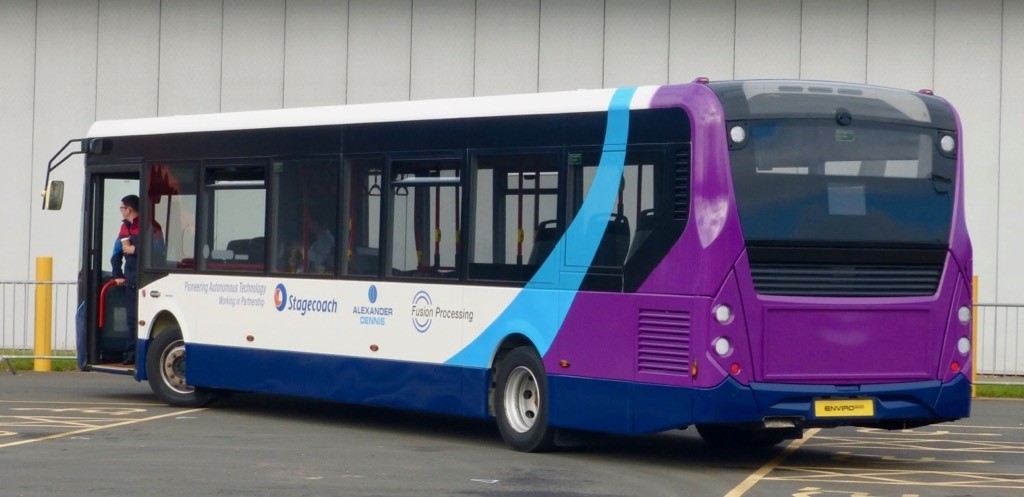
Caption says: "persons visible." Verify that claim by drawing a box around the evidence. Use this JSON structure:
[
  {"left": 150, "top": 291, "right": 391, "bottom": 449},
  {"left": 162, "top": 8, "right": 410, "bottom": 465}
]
[{"left": 111, "top": 195, "right": 167, "bottom": 364}]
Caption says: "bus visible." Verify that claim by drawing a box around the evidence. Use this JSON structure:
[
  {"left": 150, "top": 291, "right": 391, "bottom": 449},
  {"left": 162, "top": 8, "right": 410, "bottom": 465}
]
[{"left": 42, "top": 77, "right": 973, "bottom": 455}]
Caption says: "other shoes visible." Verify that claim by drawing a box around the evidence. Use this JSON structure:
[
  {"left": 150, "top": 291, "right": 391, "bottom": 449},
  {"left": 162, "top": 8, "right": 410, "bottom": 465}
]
[{"left": 122, "top": 357, "right": 135, "bottom": 365}]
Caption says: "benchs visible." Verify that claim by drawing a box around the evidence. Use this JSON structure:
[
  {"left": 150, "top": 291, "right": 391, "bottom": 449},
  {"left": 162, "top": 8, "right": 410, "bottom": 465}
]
[{"left": 468, "top": 217, "right": 631, "bottom": 293}]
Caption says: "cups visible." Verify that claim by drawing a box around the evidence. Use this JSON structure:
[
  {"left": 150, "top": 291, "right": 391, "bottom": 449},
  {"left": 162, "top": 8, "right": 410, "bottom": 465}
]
[{"left": 120, "top": 237, "right": 131, "bottom": 247}]
